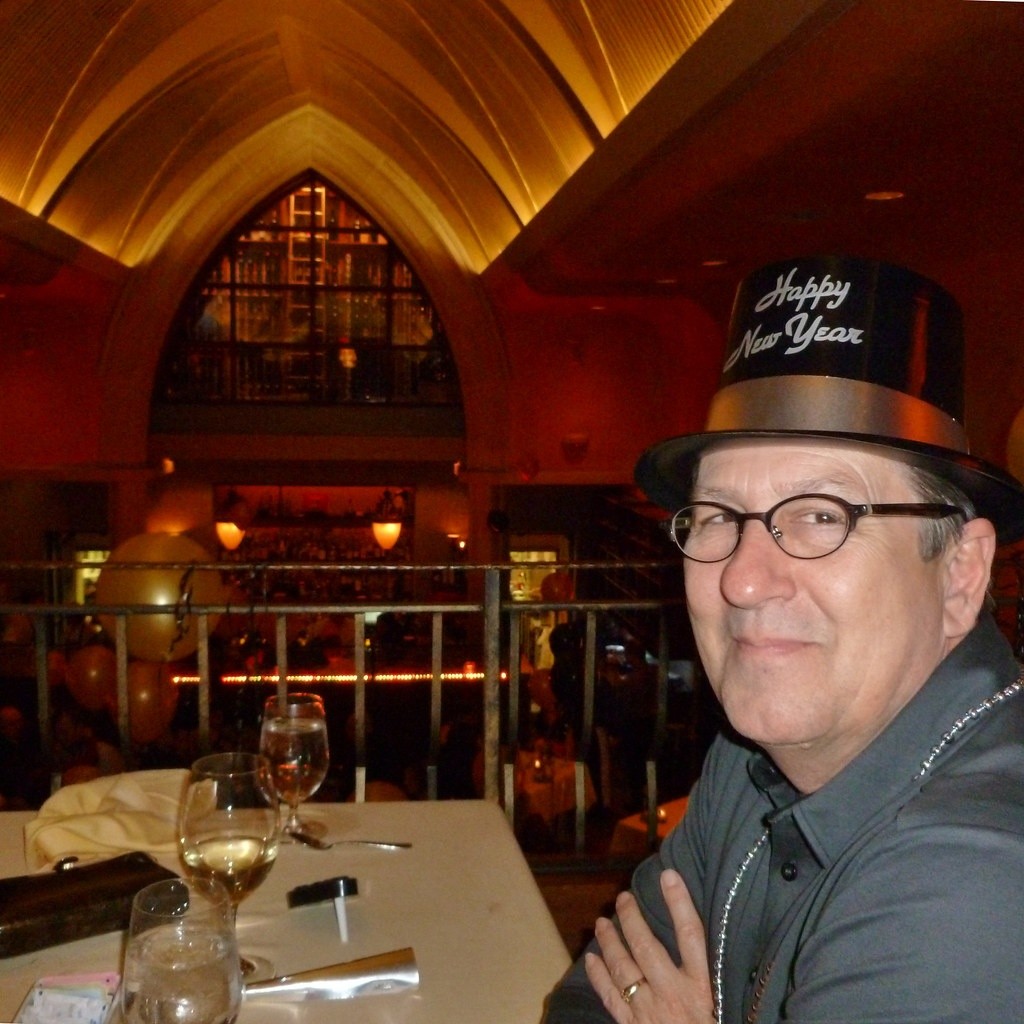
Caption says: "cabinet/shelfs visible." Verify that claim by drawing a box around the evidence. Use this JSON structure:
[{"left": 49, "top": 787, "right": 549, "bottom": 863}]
[
  {"left": 202, "top": 186, "right": 327, "bottom": 395},
  {"left": 334, "top": 197, "right": 434, "bottom": 346}
]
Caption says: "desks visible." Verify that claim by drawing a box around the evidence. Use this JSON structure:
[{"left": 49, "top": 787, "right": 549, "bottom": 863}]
[
  {"left": 0, "top": 797, "right": 572, "bottom": 1024},
  {"left": 474, "top": 751, "right": 596, "bottom": 821},
  {"left": 605, "top": 795, "right": 689, "bottom": 859}
]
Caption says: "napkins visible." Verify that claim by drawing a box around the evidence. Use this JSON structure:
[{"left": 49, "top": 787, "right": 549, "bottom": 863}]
[{"left": 22, "top": 769, "right": 217, "bottom": 875}]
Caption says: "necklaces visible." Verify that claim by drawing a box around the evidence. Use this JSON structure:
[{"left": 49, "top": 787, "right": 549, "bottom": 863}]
[{"left": 714, "top": 674, "right": 1023, "bottom": 1024}]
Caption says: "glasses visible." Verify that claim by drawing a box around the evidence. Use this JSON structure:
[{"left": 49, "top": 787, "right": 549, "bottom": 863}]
[{"left": 664, "top": 493, "right": 972, "bottom": 564}]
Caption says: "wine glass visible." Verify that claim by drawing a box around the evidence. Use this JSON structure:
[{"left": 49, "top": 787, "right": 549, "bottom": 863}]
[
  {"left": 259, "top": 693, "right": 332, "bottom": 843},
  {"left": 174, "top": 753, "right": 282, "bottom": 987}
]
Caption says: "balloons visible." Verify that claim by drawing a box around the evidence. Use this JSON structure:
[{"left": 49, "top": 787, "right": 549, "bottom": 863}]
[
  {"left": 97, "top": 530, "right": 223, "bottom": 663},
  {"left": 115, "top": 663, "right": 179, "bottom": 743},
  {"left": 66, "top": 646, "right": 116, "bottom": 707}
]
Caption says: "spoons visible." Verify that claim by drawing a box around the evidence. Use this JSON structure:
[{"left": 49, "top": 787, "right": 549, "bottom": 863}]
[{"left": 287, "top": 831, "right": 414, "bottom": 851}]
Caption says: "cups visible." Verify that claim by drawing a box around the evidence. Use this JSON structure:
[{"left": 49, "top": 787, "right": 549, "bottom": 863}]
[{"left": 122, "top": 878, "right": 241, "bottom": 1024}]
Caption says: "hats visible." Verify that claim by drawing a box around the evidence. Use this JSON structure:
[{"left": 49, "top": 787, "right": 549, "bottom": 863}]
[{"left": 633, "top": 254, "right": 1024, "bottom": 543}]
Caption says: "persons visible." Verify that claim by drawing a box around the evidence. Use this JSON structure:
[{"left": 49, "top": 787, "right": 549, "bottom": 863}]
[
  {"left": 0, "top": 600, "right": 699, "bottom": 811},
  {"left": 432, "top": 547, "right": 466, "bottom": 592},
  {"left": 223, "top": 488, "right": 409, "bottom": 599},
  {"left": 533, "top": 256, "right": 1022, "bottom": 1024}
]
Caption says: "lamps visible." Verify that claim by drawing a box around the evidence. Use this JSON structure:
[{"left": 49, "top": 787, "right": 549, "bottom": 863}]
[
  {"left": 213, "top": 485, "right": 252, "bottom": 551},
  {"left": 370, "top": 488, "right": 410, "bottom": 551}
]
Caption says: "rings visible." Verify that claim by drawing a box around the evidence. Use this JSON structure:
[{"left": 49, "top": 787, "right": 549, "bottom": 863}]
[{"left": 620, "top": 977, "right": 646, "bottom": 1000}]
